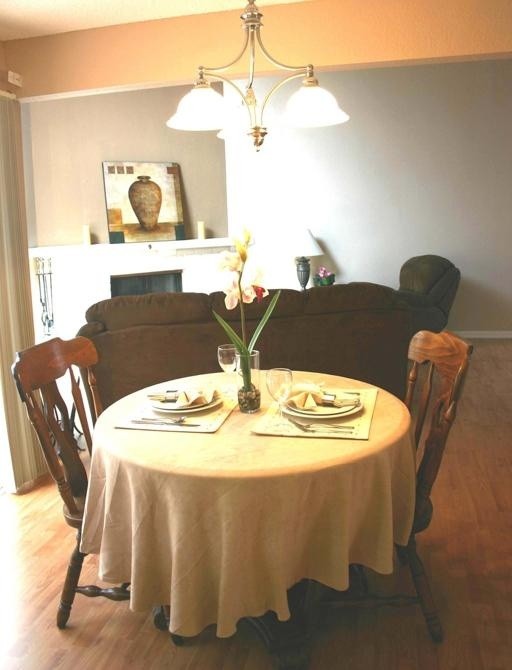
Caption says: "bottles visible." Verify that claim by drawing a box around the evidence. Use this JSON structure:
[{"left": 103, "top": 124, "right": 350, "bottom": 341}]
[{"left": 128, "top": 176, "right": 162, "bottom": 231}]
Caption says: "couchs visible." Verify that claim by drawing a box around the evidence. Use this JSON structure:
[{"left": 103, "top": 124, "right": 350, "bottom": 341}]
[{"left": 75, "top": 256, "right": 460, "bottom": 429}]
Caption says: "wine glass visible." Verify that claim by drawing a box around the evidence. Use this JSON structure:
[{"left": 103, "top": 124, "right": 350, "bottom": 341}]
[
  {"left": 266, "top": 367, "right": 294, "bottom": 430},
  {"left": 217, "top": 344, "right": 237, "bottom": 390}
]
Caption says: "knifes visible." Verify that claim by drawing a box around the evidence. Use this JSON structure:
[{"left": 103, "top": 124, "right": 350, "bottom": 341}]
[{"left": 130, "top": 420, "right": 201, "bottom": 427}]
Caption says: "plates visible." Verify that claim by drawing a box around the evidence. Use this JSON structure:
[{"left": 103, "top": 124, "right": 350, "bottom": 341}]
[
  {"left": 151, "top": 397, "right": 223, "bottom": 415},
  {"left": 280, "top": 402, "right": 364, "bottom": 420}
]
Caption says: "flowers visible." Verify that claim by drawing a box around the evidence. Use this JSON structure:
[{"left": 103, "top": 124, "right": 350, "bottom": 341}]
[
  {"left": 212, "top": 231, "right": 282, "bottom": 389},
  {"left": 311, "top": 264, "right": 337, "bottom": 288}
]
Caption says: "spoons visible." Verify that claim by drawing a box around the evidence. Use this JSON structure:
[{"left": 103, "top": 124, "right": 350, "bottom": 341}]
[{"left": 141, "top": 416, "right": 186, "bottom": 422}]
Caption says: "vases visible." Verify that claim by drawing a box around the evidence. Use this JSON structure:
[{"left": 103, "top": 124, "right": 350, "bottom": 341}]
[{"left": 234, "top": 349, "right": 263, "bottom": 413}]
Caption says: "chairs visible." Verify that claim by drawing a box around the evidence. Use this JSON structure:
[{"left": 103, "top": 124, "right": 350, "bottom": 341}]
[
  {"left": 11, "top": 335, "right": 131, "bottom": 629},
  {"left": 307, "top": 329, "right": 474, "bottom": 642}
]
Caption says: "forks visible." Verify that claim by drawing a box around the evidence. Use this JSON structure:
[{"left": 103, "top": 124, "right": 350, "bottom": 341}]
[{"left": 288, "top": 420, "right": 355, "bottom": 435}]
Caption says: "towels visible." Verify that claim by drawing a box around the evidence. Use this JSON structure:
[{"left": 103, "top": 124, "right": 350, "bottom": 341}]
[
  {"left": 286, "top": 386, "right": 322, "bottom": 415},
  {"left": 176, "top": 386, "right": 219, "bottom": 408}
]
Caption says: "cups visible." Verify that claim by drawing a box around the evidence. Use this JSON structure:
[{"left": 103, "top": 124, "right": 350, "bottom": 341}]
[{"left": 235, "top": 351, "right": 260, "bottom": 413}]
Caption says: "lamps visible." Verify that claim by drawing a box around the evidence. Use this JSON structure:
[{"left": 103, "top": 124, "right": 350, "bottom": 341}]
[{"left": 162, "top": 0, "right": 350, "bottom": 157}]
